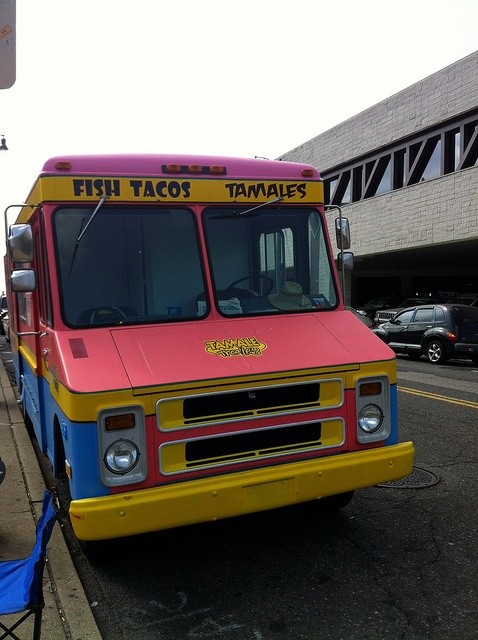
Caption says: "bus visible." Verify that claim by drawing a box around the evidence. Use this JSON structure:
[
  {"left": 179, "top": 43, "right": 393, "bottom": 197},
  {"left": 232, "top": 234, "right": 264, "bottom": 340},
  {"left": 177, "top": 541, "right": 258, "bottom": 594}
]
[{"left": 3, "top": 153, "right": 415, "bottom": 541}]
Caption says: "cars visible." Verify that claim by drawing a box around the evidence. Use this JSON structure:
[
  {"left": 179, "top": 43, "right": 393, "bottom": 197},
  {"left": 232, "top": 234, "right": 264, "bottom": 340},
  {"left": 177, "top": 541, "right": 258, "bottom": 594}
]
[
  {"left": 368, "top": 303, "right": 478, "bottom": 364},
  {"left": 446, "top": 294, "right": 478, "bottom": 308},
  {"left": 346, "top": 306, "right": 372, "bottom": 330}
]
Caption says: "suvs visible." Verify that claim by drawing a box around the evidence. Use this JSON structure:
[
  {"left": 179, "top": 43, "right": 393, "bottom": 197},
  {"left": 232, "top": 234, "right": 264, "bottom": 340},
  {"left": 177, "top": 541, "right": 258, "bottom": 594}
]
[
  {"left": 355, "top": 290, "right": 410, "bottom": 320},
  {"left": 374, "top": 292, "right": 459, "bottom": 329}
]
